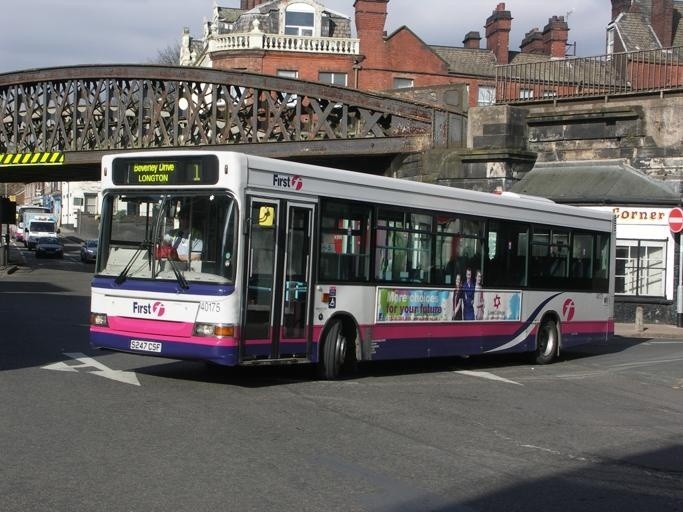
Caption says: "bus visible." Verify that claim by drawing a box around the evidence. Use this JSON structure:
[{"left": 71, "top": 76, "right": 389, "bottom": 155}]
[
  {"left": 9, "top": 205, "right": 53, "bottom": 242},
  {"left": 88, "top": 150, "right": 619, "bottom": 381}
]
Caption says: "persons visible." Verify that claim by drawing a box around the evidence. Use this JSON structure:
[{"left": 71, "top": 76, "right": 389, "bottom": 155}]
[{"left": 451, "top": 266, "right": 484, "bottom": 320}]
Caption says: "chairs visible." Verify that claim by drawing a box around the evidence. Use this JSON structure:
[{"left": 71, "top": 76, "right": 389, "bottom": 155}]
[{"left": 429, "top": 254, "right": 601, "bottom": 286}]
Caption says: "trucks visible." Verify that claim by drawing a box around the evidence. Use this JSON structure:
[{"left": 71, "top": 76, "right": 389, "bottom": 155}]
[{"left": 22, "top": 211, "right": 60, "bottom": 251}]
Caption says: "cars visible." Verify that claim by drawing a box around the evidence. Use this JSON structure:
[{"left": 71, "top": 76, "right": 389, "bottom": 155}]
[
  {"left": 35, "top": 236, "right": 64, "bottom": 258},
  {"left": 80, "top": 239, "right": 98, "bottom": 263}
]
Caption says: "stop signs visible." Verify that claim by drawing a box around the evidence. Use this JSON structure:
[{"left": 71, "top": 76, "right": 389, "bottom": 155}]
[{"left": 668, "top": 207, "right": 683, "bottom": 233}]
